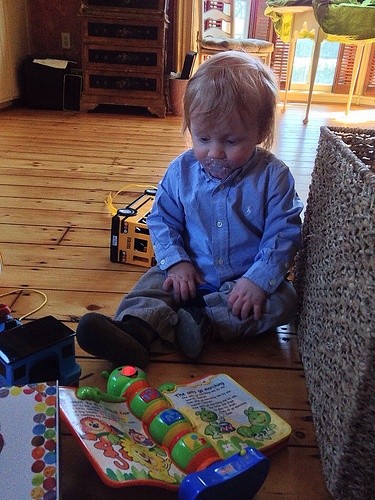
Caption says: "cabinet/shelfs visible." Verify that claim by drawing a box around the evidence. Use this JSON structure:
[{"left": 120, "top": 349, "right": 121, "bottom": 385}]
[{"left": 78, "top": 0, "right": 168, "bottom": 119}]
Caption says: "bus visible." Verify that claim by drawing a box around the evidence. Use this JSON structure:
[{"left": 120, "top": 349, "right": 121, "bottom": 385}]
[{"left": 108, "top": 185, "right": 165, "bottom": 272}]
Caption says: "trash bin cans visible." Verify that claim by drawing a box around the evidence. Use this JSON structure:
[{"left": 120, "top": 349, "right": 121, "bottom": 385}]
[{"left": 169, "top": 73, "right": 191, "bottom": 116}]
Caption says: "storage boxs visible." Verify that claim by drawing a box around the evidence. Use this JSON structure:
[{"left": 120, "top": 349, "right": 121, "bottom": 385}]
[{"left": 296, "top": 125, "right": 375, "bottom": 499}]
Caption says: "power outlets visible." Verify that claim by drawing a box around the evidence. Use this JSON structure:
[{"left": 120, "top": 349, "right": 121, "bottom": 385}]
[{"left": 61, "top": 32, "right": 71, "bottom": 49}]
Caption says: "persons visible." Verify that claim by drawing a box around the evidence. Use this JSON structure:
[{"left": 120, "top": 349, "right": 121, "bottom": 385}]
[{"left": 76, "top": 50, "right": 304, "bottom": 369}]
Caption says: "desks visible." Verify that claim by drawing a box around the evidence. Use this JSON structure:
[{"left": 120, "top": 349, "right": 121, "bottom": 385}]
[{"left": 263, "top": 3, "right": 375, "bottom": 124}]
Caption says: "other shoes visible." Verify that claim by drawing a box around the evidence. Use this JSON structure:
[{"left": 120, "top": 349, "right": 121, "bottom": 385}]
[
  {"left": 76, "top": 313, "right": 150, "bottom": 368},
  {"left": 175, "top": 307, "right": 210, "bottom": 359}
]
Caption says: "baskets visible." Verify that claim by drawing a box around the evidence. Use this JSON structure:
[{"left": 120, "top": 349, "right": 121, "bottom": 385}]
[{"left": 293, "top": 127, "right": 375, "bottom": 500}]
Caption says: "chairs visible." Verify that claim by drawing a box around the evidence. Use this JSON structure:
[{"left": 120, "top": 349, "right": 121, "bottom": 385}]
[{"left": 179, "top": 0, "right": 274, "bottom": 69}]
